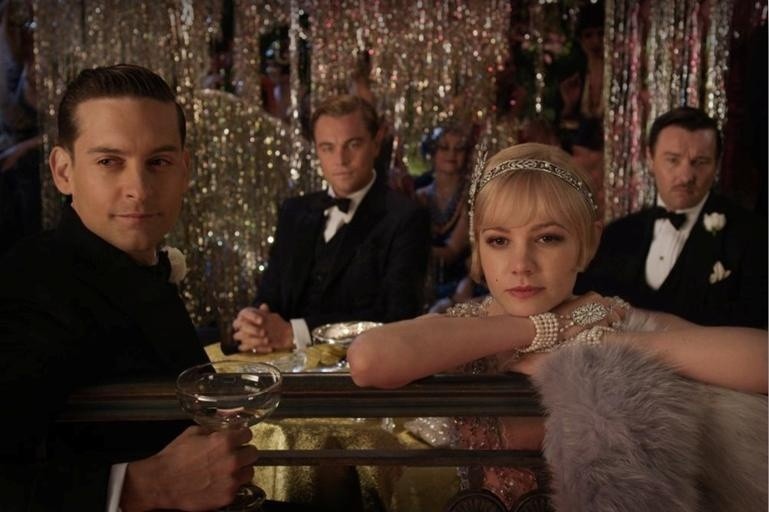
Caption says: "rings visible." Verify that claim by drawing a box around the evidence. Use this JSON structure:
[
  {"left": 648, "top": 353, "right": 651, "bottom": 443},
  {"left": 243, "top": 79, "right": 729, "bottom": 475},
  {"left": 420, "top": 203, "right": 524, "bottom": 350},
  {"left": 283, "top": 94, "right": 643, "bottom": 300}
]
[{"left": 607, "top": 295, "right": 625, "bottom": 308}]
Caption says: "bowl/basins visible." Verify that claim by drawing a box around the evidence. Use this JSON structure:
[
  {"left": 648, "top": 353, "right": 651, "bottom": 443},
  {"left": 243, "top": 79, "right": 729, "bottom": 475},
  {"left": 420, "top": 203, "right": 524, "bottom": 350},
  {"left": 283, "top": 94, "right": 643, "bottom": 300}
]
[{"left": 313, "top": 321, "right": 385, "bottom": 358}]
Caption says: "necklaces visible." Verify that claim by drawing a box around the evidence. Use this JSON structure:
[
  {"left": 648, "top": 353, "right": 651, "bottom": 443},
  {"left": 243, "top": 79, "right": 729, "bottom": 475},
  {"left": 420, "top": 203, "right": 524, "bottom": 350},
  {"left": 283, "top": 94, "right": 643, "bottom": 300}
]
[{"left": 428, "top": 175, "right": 466, "bottom": 225}]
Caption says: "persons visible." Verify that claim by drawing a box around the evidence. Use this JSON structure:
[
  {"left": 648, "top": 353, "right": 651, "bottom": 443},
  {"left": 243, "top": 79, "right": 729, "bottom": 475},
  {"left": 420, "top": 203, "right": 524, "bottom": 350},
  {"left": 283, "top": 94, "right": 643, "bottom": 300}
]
[
  {"left": 344, "top": 142, "right": 768, "bottom": 511},
  {"left": 229, "top": 93, "right": 429, "bottom": 343},
  {"left": 413, "top": 117, "right": 473, "bottom": 319},
  {"left": 573, "top": 107, "right": 768, "bottom": 332},
  {"left": 1, "top": 64, "right": 299, "bottom": 511}
]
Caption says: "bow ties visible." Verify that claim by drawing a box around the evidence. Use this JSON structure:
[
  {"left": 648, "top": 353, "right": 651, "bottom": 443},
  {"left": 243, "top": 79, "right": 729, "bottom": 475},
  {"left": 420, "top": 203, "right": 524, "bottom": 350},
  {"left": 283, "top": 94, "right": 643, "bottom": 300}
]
[
  {"left": 649, "top": 206, "right": 688, "bottom": 230},
  {"left": 323, "top": 195, "right": 351, "bottom": 213}
]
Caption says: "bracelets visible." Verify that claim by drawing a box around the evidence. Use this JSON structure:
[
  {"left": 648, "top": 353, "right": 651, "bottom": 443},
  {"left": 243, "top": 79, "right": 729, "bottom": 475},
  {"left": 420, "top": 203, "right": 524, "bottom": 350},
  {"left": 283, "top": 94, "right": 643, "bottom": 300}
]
[{"left": 512, "top": 312, "right": 558, "bottom": 353}]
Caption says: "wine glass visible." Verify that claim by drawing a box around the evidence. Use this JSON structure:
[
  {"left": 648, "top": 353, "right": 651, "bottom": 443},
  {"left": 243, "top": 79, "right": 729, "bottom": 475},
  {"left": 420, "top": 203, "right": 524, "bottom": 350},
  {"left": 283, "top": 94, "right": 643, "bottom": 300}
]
[{"left": 175, "top": 360, "right": 283, "bottom": 509}]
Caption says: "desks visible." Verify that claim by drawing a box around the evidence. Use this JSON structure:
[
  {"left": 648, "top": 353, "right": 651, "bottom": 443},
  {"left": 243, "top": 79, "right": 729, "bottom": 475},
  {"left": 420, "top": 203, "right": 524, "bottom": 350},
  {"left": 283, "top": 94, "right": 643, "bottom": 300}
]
[{"left": 205, "top": 341, "right": 469, "bottom": 510}]
